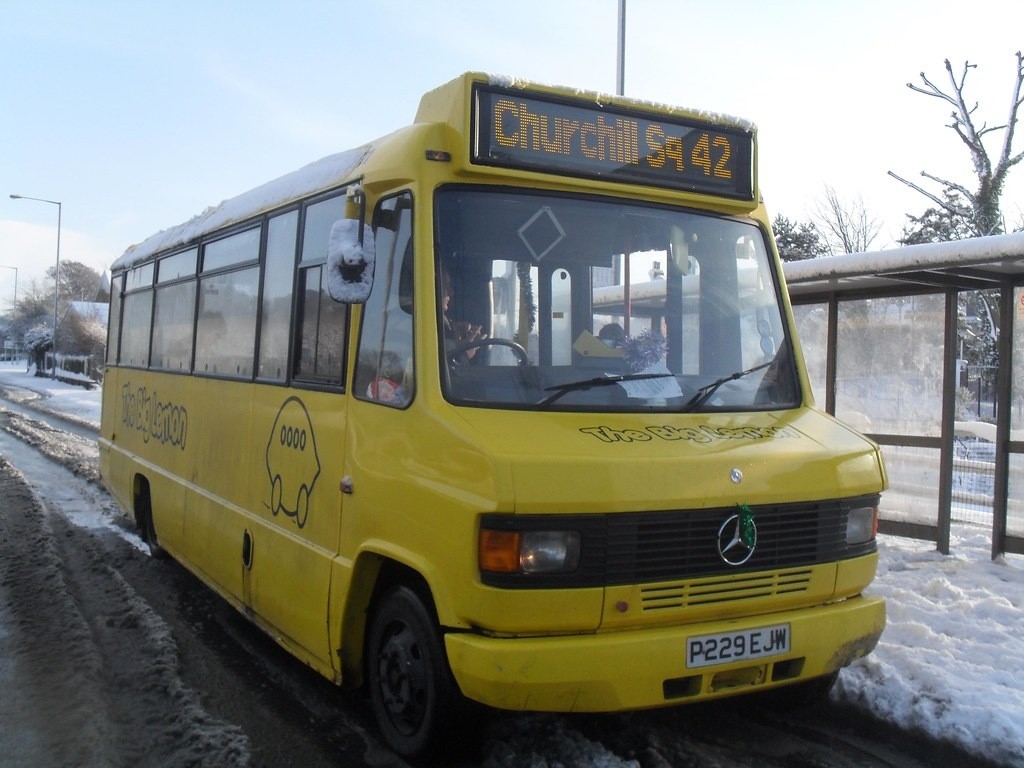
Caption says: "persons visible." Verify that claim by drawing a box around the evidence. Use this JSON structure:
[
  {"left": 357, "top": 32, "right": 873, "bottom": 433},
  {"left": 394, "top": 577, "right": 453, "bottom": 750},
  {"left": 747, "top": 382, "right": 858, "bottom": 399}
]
[
  {"left": 164, "top": 309, "right": 228, "bottom": 369},
  {"left": 387, "top": 265, "right": 488, "bottom": 398}
]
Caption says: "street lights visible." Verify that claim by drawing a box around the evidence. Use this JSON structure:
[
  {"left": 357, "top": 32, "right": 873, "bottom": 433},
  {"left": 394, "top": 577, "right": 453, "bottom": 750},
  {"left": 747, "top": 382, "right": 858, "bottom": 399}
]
[{"left": 9, "top": 193, "right": 62, "bottom": 380}]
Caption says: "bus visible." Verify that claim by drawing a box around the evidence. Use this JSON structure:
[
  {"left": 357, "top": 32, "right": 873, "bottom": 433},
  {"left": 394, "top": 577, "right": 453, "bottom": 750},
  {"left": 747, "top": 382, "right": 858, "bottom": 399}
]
[{"left": 96, "top": 73, "right": 889, "bottom": 768}]
